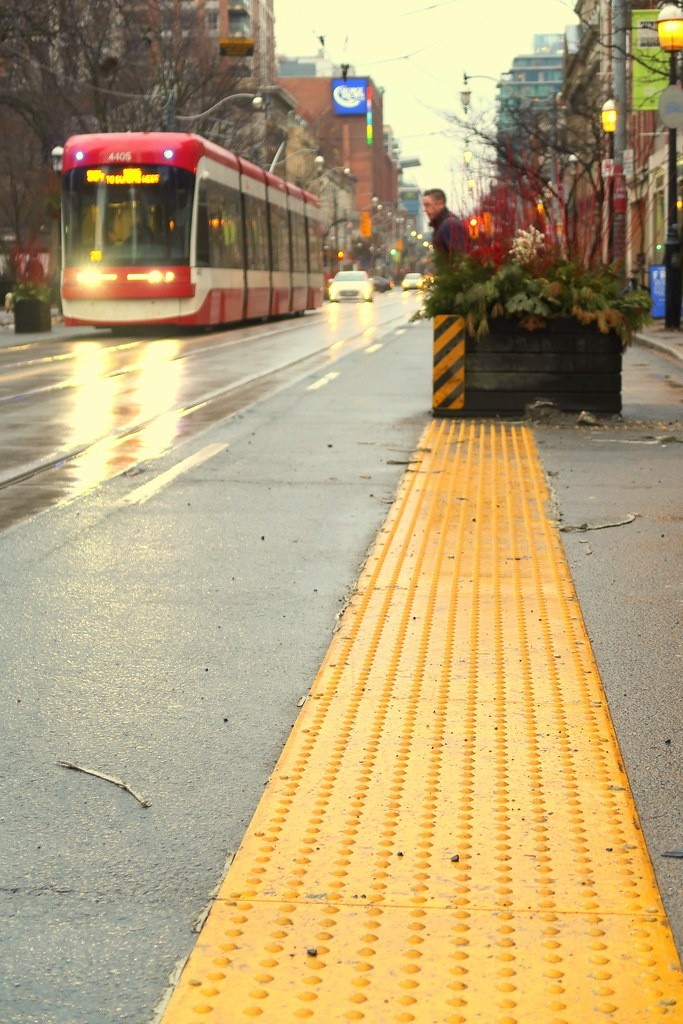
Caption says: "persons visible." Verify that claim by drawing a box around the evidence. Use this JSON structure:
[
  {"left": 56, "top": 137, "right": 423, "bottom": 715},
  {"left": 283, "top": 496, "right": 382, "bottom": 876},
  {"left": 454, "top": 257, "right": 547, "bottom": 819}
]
[
  {"left": 22, "top": 251, "right": 44, "bottom": 287},
  {"left": 423, "top": 189, "right": 475, "bottom": 275}
]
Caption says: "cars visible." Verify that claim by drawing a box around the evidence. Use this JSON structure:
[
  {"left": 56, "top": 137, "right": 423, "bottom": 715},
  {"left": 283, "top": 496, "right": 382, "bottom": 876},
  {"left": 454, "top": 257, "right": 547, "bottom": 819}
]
[
  {"left": 375, "top": 277, "right": 391, "bottom": 292},
  {"left": 327, "top": 271, "right": 375, "bottom": 302},
  {"left": 402, "top": 272, "right": 424, "bottom": 291}
]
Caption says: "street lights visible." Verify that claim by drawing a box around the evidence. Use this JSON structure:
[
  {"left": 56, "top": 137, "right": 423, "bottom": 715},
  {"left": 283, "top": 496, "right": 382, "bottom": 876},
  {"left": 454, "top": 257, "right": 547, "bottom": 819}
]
[{"left": 461, "top": 72, "right": 565, "bottom": 249}]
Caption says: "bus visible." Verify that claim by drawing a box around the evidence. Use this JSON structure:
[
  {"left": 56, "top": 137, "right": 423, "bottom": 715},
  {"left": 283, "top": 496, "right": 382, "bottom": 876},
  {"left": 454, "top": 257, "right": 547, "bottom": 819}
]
[{"left": 52, "top": 130, "right": 326, "bottom": 334}]
